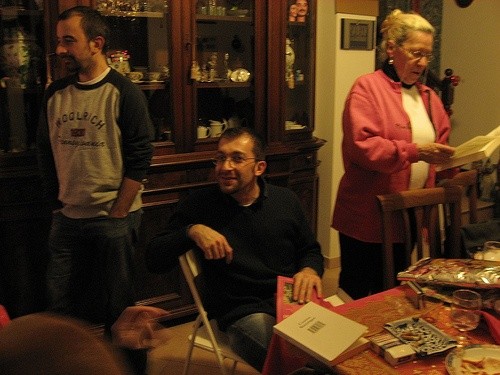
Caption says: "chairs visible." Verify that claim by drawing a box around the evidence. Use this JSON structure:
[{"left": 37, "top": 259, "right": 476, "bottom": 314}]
[
  {"left": 376, "top": 187, "right": 462, "bottom": 290},
  {"left": 176, "top": 247, "right": 255, "bottom": 375},
  {"left": 438, "top": 169, "right": 479, "bottom": 244}
]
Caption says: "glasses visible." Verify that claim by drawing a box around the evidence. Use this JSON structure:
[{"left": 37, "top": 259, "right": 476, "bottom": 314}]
[
  {"left": 395, "top": 40, "right": 434, "bottom": 63},
  {"left": 210, "top": 152, "right": 257, "bottom": 166}
]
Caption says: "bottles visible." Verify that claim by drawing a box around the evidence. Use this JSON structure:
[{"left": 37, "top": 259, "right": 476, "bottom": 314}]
[
  {"left": 198, "top": 0, "right": 226, "bottom": 16},
  {"left": 285, "top": 38, "right": 295, "bottom": 82},
  {"left": 2, "top": 29, "right": 40, "bottom": 70}
]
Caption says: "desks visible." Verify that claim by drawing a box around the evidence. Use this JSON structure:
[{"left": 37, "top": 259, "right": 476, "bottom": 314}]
[{"left": 260, "top": 282, "right": 500, "bottom": 375}]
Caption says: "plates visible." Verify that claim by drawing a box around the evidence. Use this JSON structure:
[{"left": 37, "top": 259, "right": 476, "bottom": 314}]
[{"left": 444, "top": 342, "right": 500, "bottom": 375}]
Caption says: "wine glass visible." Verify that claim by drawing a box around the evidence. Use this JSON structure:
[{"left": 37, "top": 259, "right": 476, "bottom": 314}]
[{"left": 449, "top": 290, "right": 482, "bottom": 348}]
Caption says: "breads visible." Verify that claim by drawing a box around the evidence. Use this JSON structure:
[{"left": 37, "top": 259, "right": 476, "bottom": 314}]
[{"left": 460, "top": 355, "right": 500, "bottom": 375}]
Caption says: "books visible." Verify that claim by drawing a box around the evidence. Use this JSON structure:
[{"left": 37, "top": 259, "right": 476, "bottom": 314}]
[
  {"left": 272, "top": 301, "right": 371, "bottom": 368},
  {"left": 433, "top": 126, "right": 500, "bottom": 172},
  {"left": 276, "top": 275, "right": 321, "bottom": 324}
]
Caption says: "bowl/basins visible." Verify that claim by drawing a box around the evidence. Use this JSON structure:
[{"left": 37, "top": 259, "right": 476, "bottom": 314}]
[{"left": 229, "top": 8, "right": 250, "bottom": 17}]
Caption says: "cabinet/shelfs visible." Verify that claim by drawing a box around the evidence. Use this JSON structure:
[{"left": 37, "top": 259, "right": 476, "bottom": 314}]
[{"left": 0, "top": 0, "right": 329, "bottom": 328}]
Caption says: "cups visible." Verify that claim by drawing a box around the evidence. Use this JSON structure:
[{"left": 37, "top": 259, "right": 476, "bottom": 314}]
[
  {"left": 126, "top": 67, "right": 171, "bottom": 83},
  {"left": 197, "top": 114, "right": 249, "bottom": 139}
]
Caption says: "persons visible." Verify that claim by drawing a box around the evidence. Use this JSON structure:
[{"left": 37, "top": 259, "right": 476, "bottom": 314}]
[
  {"left": 36, "top": 5, "right": 153, "bottom": 342},
  {"left": 0, "top": 305, "right": 168, "bottom": 375},
  {"left": 161, "top": 127, "right": 325, "bottom": 375},
  {"left": 331, "top": 8, "right": 455, "bottom": 302}
]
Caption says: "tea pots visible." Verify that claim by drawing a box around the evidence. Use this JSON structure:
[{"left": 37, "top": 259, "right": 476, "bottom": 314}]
[{"left": 106, "top": 50, "right": 131, "bottom": 77}]
